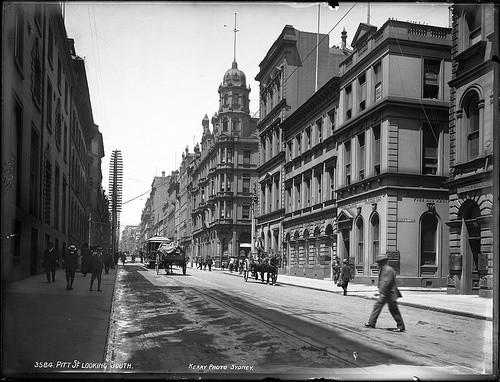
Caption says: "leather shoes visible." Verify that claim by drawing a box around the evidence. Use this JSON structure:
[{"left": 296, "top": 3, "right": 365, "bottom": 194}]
[
  {"left": 365, "top": 322, "right": 375, "bottom": 328},
  {"left": 393, "top": 326, "right": 405, "bottom": 333}
]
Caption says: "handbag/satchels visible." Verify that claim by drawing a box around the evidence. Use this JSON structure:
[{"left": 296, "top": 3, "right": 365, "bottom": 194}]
[{"left": 337, "top": 279, "right": 346, "bottom": 287}]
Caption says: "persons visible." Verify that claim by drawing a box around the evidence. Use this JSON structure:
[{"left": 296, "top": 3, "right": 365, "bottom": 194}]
[
  {"left": 43, "top": 242, "right": 59, "bottom": 283},
  {"left": 197, "top": 258, "right": 204, "bottom": 270},
  {"left": 138, "top": 247, "right": 146, "bottom": 263},
  {"left": 63, "top": 244, "right": 79, "bottom": 290},
  {"left": 340, "top": 258, "right": 352, "bottom": 295},
  {"left": 104, "top": 250, "right": 111, "bottom": 273},
  {"left": 331, "top": 256, "right": 341, "bottom": 284},
  {"left": 80, "top": 243, "right": 90, "bottom": 278},
  {"left": 191, "top": 257, "right": 194, "bottom": 267},
  {"left": 363, "top": 254, "right": 406, "bottom": 332},
  {"left": 121, "top": 252, "right": 127, "bottom": 266},
  {"left": 203, "top": 256, "right": 213, "bottom": 271},
  {"left": 89, "top": 245, "right": 103, "bottom": 291},
  {"left": 257, "top": 237, "right": 265, "bottom": 264},
  {"left": 195, "top": 256, "right": 198, "bottom": 267}
]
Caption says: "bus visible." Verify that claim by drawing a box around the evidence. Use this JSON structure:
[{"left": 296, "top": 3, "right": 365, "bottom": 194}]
[{"left": 145, "top": 236, "right": 171, "bottom": 268}]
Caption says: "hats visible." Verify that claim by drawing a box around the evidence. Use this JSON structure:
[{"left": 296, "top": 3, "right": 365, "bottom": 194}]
[
  {"left": 343, "top": 259, "right": 348, "bottom": 263},
  {"left": 68, "top": 244, "right": 77, "bottom": 251},
  {"left": 375, "top": 255, "right": 390, "bottom": 262}
]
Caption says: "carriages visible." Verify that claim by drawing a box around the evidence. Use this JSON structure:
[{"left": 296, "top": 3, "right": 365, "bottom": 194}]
[
  {"left": 244, "top": 251, "right": 279, "bottom": 285},
  {"left": 155, "top": 239, "right": 186, "bottom": 274}
]
